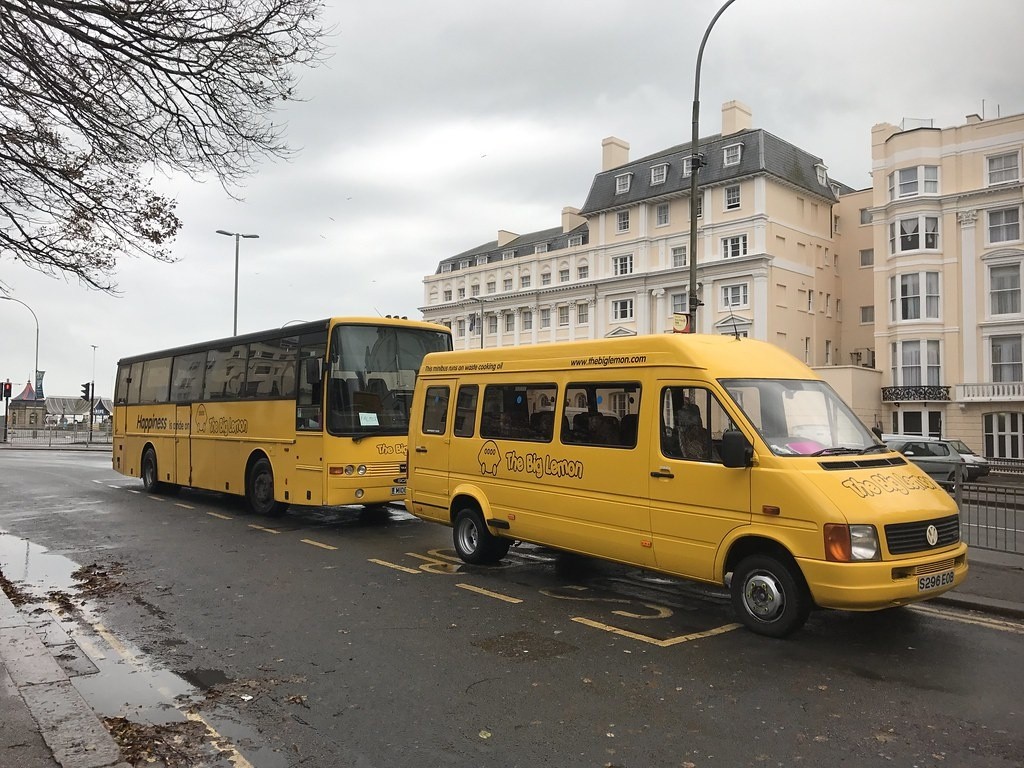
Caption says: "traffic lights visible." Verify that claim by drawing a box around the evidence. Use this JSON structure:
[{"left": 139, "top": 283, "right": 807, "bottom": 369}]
[
  {"left": 4, "top": 383, "right": 11, "bottom": 397},
  {"left": 80, "top": 382, "right": 90, "bottom": 402}
]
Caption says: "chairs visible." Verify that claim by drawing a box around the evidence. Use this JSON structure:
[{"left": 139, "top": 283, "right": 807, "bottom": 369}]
[
  {"left": 314, "top": 378, "right": 399, "bottom": 410},
  {"left": 484, "top": 404, "right": 750, "bottom": 466}
]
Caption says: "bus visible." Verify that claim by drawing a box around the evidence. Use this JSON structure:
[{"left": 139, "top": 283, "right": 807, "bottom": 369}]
[
  {"left": 405, "top": 333, "right": 969, "bottom": 638},
  {"left": 111, "top": 314, "right": 454, "bottom": 521}
]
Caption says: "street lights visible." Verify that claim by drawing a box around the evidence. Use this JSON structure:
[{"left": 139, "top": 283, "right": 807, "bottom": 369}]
[
  {"left": 216, "top": 229, "right": 260, "bottom": 336},
  {"left": 469, "top": 296, "right": 495, "bottom": 350},
  {"left": 91, "top": 344, "right": 98, "bottom": 384}
]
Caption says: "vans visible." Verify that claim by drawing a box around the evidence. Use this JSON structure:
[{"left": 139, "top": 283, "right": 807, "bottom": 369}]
[{"left": 881, "top": 434, "right": 990, "bottom": 482}]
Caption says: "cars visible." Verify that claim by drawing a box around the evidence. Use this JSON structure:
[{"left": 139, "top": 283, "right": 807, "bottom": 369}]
[{"left": 884, "top": 438, "right": 969, "bottom": 493}]
